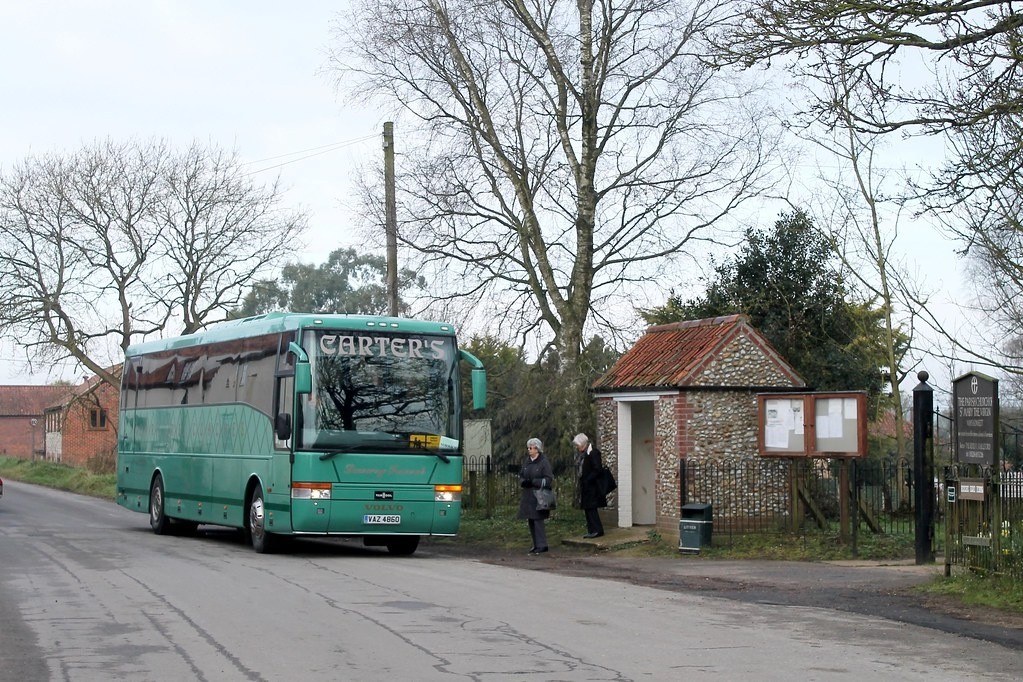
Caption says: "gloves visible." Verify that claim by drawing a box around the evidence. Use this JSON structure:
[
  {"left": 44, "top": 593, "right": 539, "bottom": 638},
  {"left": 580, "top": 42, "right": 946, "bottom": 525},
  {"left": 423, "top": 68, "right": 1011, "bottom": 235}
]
[{"left": 521, "top": 480, "right": 532, "bottom": 488}]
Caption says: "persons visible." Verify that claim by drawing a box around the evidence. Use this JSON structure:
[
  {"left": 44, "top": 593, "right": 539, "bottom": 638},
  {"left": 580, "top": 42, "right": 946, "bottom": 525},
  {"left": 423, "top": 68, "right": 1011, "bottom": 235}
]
[
  {"left": 519, "top": 437, "right": 555, "bottom": 556},
  {"left": 571, "top": 433, "right": 607, "bottom": 538}
]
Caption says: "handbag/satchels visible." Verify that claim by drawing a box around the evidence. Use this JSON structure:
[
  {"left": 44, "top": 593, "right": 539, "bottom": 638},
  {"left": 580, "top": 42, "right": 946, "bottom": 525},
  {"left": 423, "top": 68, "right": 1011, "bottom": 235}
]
[
  {"left": 594, "top": 467, "right": 617, "bottom": 497},
  {"left": 534, "top": 489, "right": 556, "bottom": 511}
]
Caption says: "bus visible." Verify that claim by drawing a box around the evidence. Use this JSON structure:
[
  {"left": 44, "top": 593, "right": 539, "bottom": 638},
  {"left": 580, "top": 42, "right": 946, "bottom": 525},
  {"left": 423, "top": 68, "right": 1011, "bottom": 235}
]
[{"left": 113, "top": 311, "right": 487, "bottom": 556}]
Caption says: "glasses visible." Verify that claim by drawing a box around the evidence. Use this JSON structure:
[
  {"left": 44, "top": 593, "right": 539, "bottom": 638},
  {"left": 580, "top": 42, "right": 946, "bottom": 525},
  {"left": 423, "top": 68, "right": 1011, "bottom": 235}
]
[{"left": 527, "top": 446, "right": 538, "bottom": 449}]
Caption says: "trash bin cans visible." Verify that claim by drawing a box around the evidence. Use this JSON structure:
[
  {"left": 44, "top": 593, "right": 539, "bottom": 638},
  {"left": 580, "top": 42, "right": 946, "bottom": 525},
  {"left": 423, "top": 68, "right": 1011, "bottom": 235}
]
[{"left": 677, "top": 503, "right": 714, "bottom": 558}]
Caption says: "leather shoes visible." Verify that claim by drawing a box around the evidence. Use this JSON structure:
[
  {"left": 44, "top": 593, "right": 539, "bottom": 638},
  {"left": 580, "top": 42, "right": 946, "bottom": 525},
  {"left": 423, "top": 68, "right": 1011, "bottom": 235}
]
[
  {"left": 531, "top": 547, "right": 548, "bottom": 553},
  {"left": 584, "top": 533, "right": 605, "bottom": 538}
]
